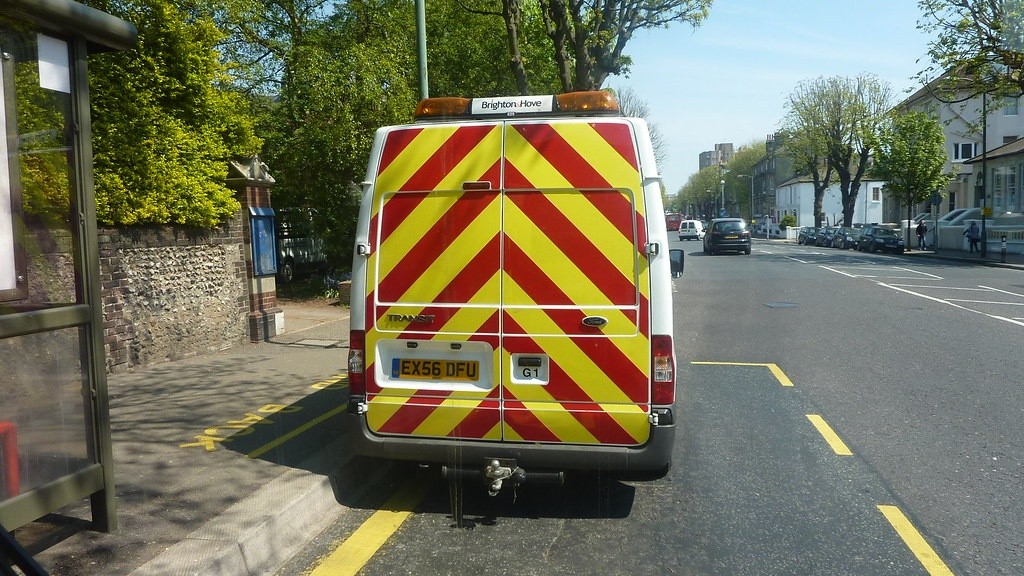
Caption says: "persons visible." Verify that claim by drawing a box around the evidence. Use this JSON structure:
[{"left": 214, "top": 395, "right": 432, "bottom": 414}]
[
  {"left": 962, "top": 221, "right": 979, "bottom": 253},
  {"left": 915, "top": 219, "right": 928, "bottom": 251}
]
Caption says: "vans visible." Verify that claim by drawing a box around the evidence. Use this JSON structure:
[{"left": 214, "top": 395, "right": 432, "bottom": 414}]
[
  {"left": 678, "top": 220, "right": 707, "bottom": 241},
  {"left": 938, "top": 207, "right": 1007, "bottom": 226},
  {"left": 347, "top": 89, "right": 685, "bottom": 498}
]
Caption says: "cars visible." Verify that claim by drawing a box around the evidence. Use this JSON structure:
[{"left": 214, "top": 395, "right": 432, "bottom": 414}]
[{"left": 798, "top": 225, "right": 904, "bottom": 255}]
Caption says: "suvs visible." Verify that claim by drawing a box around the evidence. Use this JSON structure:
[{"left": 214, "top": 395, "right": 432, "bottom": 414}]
[{"left": 703, "top": 217, "right": 752, "bottom": 256}]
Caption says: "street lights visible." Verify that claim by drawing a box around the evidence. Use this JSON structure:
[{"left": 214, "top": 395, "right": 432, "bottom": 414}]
[
  {"left": 720, "top": 179, "right": 726, "bottom": 210},
  {"left": 737, "top": 174, "right": 755, "bottom": 236},
  {"left": 943, "top": 77, "right": 987, "bottom": 256}
]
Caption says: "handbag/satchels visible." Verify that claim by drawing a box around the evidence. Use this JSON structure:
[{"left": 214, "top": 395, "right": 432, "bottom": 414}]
[{"left": 966, "top": 231, "right": 973, "bottom": 238}]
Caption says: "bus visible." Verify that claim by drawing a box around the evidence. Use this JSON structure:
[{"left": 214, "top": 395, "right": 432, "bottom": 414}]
[{"left": 665, "top": 212, "right": 685, "bottom": 231}]
